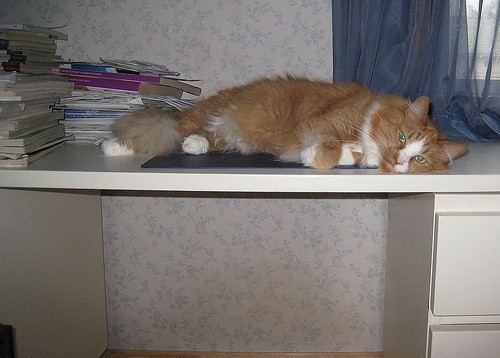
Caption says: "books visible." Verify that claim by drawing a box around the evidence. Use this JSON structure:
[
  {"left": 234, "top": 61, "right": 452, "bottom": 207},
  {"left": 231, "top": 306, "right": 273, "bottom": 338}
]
[
  {"left": 76, "top": 56, "right": 202, "bottom": 146},
  {"left": 0, "top": 22, "right": 75, "bottom": 168}
]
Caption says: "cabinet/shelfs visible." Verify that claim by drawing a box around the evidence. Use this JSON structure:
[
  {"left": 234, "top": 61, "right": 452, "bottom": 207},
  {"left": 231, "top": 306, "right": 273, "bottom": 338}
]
[{"left": 0, "top": 144, "right": 499, "bottom": 358}]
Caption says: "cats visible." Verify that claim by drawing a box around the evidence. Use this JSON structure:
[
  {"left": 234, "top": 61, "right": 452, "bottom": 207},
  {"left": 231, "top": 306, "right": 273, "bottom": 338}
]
[{"left": 100, "top": 70, "right": 467, "bottom": 173}]
